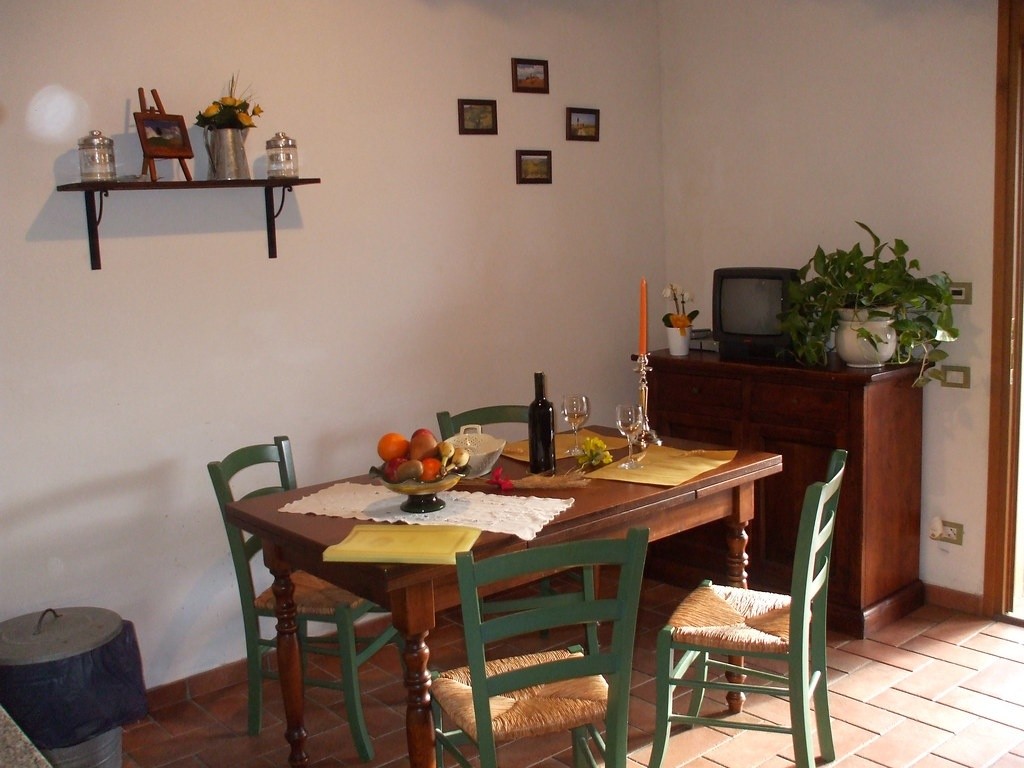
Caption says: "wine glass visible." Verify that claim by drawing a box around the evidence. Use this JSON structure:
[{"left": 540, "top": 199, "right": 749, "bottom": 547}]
[
  {"left": 561, "top": 395, "right": 589, "bottom": 456},
  {"left": 615, "top": 404, "right": 645, "bottom": 470}
]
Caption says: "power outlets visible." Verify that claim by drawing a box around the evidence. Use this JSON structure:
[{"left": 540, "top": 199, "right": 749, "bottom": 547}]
[
  {"left": 930, "top": 518, "right": 966, "bottom": 544},
  {"left": 943, "top": 367, "right": 971, "bottom": 390}
]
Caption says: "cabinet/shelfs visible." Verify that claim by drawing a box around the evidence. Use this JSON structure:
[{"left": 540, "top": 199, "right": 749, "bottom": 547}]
[{"left": 631, "top": 349, "right": 938, "bottom": 640}]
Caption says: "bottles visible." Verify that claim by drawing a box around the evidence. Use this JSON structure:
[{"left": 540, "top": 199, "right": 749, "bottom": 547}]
[
  {"left": 266, "top": 133, "right": 300, "bottom": 180},
  {"left": 526, "top": 372, "right": 556, "bottom": 478},
  {"left": 78, "top": 130, "right": 117, "bottom": 184}
]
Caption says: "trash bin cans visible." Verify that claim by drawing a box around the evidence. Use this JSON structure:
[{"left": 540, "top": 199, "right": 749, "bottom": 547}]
[{"left": 0, "top": 607, "right": 125, "bottom": 768}]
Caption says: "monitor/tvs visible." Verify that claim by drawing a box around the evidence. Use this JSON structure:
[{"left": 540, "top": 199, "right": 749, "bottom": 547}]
[{"left": 712, "top": 266, "right": 802, "bottom": 366}]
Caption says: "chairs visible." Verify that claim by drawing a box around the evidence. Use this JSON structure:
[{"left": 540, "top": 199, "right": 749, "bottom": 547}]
[
  {"left": 208, "top": 435, "right": 410, "bottom": 763},
  {"left": 435, "top": 403, "right": 554, "bottom": 642},
  {"left": 643, "top": 447, "right": 851, "bottom": 767},
  {"left": 429, "top": 525, "right": 651, "bottom": 768}
]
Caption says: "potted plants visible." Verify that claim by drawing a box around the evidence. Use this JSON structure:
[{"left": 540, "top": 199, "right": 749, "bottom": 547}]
[{"left": 778, "top": 221, "right": 960, "bottom": 370}]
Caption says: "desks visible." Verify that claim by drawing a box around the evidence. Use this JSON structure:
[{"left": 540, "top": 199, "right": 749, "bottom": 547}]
[{"left": 225, "top": 428, "right": 784, "bottom": 767}]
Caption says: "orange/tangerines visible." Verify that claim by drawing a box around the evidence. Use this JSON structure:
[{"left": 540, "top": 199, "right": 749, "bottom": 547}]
[{"left": 378, "top": 433, "right": 410, "bottom": 460}]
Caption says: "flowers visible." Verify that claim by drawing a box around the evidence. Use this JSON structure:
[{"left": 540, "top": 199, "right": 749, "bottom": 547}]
[
  {"left": 661, "top": 281, "right": 700, "bottom": 325},
  {"left": 195, "top": 77, "right": 266, "bottom": 132}
]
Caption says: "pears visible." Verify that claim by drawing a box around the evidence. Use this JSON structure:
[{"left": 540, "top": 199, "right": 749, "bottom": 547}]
[{"left": 407, "top": 434, "right": 439, "bottom": 462}]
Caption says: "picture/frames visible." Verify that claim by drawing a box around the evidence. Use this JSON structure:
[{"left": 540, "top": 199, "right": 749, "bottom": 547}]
[
  {"left": 516, "top": 149, "right": 553, "bottom": 185},
  {"left": 134, "top": 113, "right": 191, "bottom": 155},
  {"left": 458, "top": 98, "right": 497, "bottom": 135},
  {"left": 565, "top": 108, "right": 601, "bottom": 142},
  {"left": 510, "top": 57, "right": 551, "bottom": 93}
]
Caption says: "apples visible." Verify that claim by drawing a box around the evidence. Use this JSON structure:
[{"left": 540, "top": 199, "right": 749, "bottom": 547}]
[{"left": 384, "top": 428, "right": 442, "bottom": 484}]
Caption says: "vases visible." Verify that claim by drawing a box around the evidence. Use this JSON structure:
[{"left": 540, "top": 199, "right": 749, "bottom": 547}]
[
  {"left": 667, "top": 322, "right": 692, "bottom": 355},
  {"left": 196, "top": 125, "right": 251, "bottom": 178}
]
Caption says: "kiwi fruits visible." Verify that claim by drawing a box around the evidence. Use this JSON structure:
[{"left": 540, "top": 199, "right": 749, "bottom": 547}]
[{"left": 397, "top": 460, "right": 423, "bottom": 480}]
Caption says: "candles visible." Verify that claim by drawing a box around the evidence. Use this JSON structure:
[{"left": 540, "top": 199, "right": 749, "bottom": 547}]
[{"left": 638, "top": 275, "right": 647, "bottom": 358}]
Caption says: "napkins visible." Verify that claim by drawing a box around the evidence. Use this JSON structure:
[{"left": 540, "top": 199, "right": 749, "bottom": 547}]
[
  {"left": 585, "top": 443, "right": 738, "bottom": 486},
  {"left": 318, "top": 523, "right": 479, "bottom": 566},
  {"left": 497, "top": 424, "right": 630, "bottom": 466}
]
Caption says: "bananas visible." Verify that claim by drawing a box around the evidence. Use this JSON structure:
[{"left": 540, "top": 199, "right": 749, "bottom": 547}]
[{"left": 439, "top": 442, "right": 469, "bottom": 474}]
